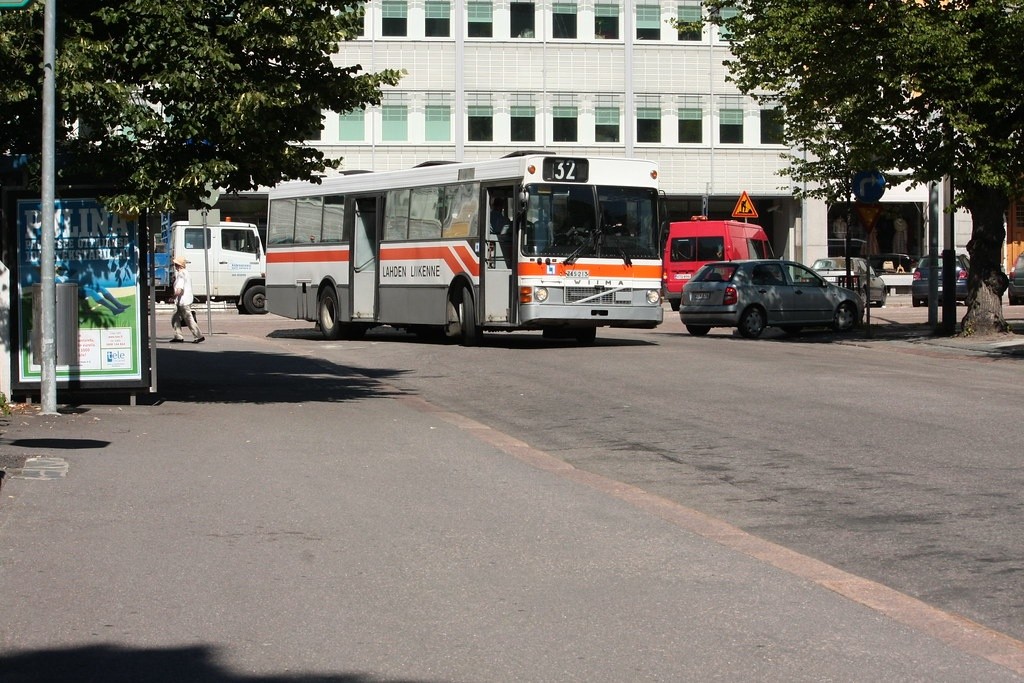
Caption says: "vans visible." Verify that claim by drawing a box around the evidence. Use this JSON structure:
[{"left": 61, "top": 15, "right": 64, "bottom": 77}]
[{"left": 663, "top": 215, "right": 774, "bottom": 311}]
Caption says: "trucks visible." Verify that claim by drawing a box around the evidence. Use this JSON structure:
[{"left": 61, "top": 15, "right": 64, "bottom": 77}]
[{"left": 147, "top": 206, "right": 266, "bottom": 316}]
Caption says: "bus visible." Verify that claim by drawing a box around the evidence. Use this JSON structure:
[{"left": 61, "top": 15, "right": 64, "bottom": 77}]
[{"left": 265, "top": 149, "right": 665, "bottom": 347}]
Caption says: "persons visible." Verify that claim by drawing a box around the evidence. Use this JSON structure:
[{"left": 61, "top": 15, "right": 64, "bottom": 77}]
[
  {"left": 169, "top": 256, "right": 206, "bottom": 343},
  {"left": 859, "top": 227, "right": 880, "bottom": 255},
  {"left": 833, "top": 218, "right": 847, "bottom": 239},
  {"left": 893, "top": 217, "right": 908, "bottom": 253}
]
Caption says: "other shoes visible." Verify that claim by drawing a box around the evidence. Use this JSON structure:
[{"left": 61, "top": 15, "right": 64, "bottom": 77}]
[
  {"left": 169, "top": 337, "right": 184, "bottom": 343},
  {"left": 192, "top": 337, "right": 205, "bottom": 343}
]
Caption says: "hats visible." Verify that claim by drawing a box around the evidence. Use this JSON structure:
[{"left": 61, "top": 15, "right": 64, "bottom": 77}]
[{"left": 173, "top": 256, "right": 186, "bottom": 267}]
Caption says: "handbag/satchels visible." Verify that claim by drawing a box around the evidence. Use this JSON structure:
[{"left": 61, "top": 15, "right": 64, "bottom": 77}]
[{"left": 172, "top": 311, "right": 197, "bottom": 328}]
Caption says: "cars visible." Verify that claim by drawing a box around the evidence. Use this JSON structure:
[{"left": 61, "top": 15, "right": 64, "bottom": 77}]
[
  {"left": 1008, "top": 251, "right": 1024, "bottom": 305},
  {"left": 814, "top": 258, "right": 888, "bottom": 305},
  {"left": 906, "top": 253, "right": 972, "bottom": 310},
  {"left": 680, "top": 259, "right": 866, "bottom": 335}
]
